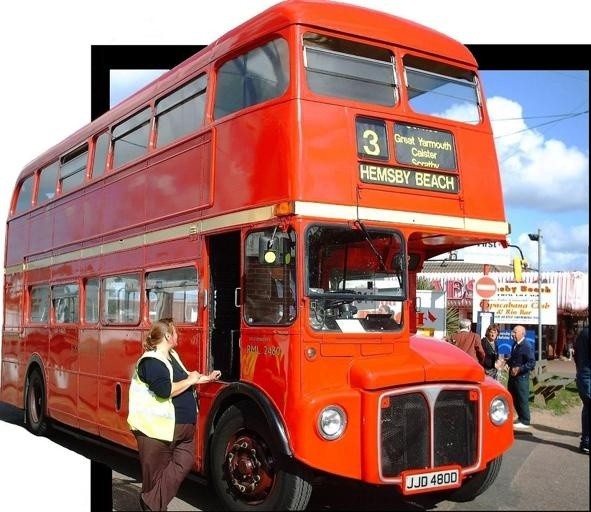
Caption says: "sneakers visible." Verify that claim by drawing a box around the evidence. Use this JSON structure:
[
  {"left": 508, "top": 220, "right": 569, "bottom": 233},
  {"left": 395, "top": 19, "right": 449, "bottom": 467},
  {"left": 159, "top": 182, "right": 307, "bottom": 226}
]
[
  {"left": 579, "top": 446, "right": 589, "bottom": 453},
  {"left": 514, "top": 421, "right": 529, "bottom": 429},
  {"left": 137, "top": 498, "right": 151, "bottom": 512}
]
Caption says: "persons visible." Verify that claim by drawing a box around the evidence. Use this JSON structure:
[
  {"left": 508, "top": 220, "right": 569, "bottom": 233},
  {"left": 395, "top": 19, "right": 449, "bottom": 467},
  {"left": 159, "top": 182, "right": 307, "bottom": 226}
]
[
  {"left": 503, "top": 325, "right": 537, "bottom": 429},
  {"left": 126, "top": 318, "right": 222, "bottom": 512},
  {"left": 573, "top": 325, "right": 591, "bottom": 455},
  {"left": 246, "top": 262, "right": 295, "bottom": 325},
  {"left": 479, "top": 324, "right": 507, "bottom": 382},
  {"left": 444, "top": 318, "right": 486, "bottom": 365}
]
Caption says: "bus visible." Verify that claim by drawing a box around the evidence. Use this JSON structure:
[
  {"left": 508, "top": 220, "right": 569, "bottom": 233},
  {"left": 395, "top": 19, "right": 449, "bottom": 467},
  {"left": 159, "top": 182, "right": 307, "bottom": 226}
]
[{"left": 0, "top": 0, "right": 516, "bottom": 512}]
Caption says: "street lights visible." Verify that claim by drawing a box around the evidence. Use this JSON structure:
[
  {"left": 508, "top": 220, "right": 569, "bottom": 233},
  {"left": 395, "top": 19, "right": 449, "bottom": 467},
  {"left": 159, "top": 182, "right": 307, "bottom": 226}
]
[{"left": 528, "top": 230, "right": 543, "bottom": 362}]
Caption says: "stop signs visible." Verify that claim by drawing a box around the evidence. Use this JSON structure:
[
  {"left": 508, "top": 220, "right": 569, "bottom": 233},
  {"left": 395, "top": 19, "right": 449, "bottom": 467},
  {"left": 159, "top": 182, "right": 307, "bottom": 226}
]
[{"left": 475, "top": 277, "right": 496, "bottom": 298}]
